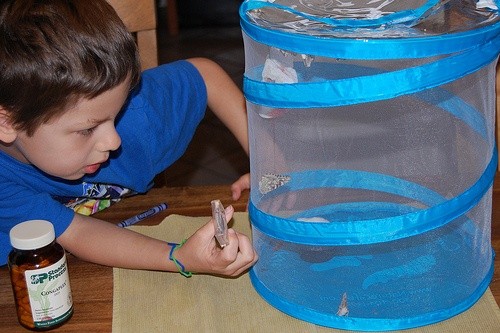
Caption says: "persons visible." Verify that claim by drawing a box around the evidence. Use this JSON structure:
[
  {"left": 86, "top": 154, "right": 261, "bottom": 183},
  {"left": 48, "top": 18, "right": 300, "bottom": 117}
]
[{"left": 0, "top": 0, "right": 296, "bottom": 278}]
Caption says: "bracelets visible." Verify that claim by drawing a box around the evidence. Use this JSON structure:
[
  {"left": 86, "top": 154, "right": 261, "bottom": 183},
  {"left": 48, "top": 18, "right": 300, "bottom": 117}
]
[{"left": 167, "top": 239, "right": 192, "bottom": 277}]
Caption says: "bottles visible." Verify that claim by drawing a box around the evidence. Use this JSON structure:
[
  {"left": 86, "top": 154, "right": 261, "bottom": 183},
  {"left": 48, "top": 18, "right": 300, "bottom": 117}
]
[{"left": 9, "top": 219, "right": 73, "bottom": 330}]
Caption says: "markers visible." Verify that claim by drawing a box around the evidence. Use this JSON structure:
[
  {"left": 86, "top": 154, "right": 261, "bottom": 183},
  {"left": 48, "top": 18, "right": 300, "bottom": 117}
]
[{"left": 119, "top": 203, "right": 168, "bottom": 228}]
